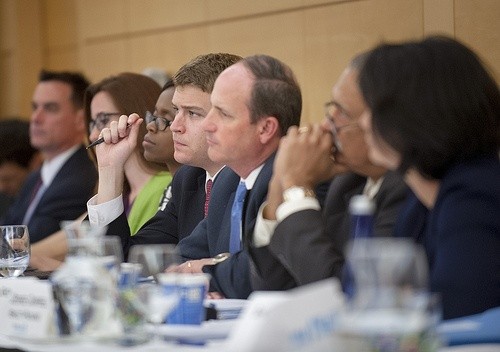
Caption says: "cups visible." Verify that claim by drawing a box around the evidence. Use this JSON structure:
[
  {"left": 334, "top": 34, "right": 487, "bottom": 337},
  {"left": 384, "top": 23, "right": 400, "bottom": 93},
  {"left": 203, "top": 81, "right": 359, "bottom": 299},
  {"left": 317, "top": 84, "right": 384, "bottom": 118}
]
[
  {"left": 334, "top": 238, "right": 445, "bottom": 351},
  {"left": 157, "top": 271, "right": 211, "bottom": 344},
  {"left": 117, "top": 262, "right": 142, "bottom": 295},
  {"left": 128, "top": 244, "right": 182, "bottom": 287},
  {"left": 66, "top": 236, "right": 124, "bottom": 281},
  {"left": 0, "top": 225, "right": 30, "bottom": 278}
]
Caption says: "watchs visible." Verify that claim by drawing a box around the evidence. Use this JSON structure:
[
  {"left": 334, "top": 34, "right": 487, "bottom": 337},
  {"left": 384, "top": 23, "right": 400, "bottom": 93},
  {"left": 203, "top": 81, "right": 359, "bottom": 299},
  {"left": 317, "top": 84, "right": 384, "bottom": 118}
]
[
  {"left": 283, "top": 184, "right": 317, "bottom": 202},
  {"left": 209, "top": 252, "right": 230, "bottom": 265}
]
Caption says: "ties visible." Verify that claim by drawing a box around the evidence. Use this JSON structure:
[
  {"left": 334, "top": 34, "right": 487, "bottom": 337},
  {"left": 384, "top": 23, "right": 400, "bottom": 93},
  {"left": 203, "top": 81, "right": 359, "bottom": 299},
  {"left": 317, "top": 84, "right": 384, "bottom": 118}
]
[
  {"left": 346, "top": 215, "right": 370, "bottom": 297},
  {"left": 28, "top": 178, "right": 43, "bottom": 208},
  {"left": 204, "top": 180, "right": 212, "bottom": 217},
  {"left": 229, "top": 182, "right": 247, "bottom": 255}
]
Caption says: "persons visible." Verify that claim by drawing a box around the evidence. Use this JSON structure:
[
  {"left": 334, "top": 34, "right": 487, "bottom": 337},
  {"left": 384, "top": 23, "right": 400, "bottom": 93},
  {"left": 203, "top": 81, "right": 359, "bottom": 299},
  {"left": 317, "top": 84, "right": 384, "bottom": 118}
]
[
  {"left": 143, "top": 77, "right": 184, "bottom": 217},
  {"left": 10, "top": 71, "right": 173, "bottom": 271},
  {"left": 357, "top": 35, "right": 500, "bottom": 320},
  {"left": 245, "top": 49, "right": 410, "bottom": 291},
  {"left": 165, "top": 54, "right": 302, "bottom": 300},
  {"left": 85, "top": 53, "right": 244, "bottom": 269},
  {"left": 0, "top": 68, "right": 99, "bottom": 260}
]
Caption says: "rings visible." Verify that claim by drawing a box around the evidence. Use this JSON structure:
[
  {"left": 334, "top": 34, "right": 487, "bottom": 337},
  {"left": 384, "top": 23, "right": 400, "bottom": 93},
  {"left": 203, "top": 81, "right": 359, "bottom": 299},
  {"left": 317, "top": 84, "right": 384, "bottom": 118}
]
[
  {"left": 299, "top": 127, "right": 311, "bottom": 133},
  {"left": 188, "top": 262, "right": 191, "bottom": 267}
]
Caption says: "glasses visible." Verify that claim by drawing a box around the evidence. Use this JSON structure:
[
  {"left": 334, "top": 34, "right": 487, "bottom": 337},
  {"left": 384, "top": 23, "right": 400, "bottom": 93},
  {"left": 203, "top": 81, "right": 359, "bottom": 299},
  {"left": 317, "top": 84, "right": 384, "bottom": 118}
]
[
  {"left": 89, "top": 112, "right": 119, "bottom": 134},
  {"left": 145, "top": 111, "right": 173, "bottom": 131},
  {"left": 324, "top": 102, "right": 358, "bottom": 136}
]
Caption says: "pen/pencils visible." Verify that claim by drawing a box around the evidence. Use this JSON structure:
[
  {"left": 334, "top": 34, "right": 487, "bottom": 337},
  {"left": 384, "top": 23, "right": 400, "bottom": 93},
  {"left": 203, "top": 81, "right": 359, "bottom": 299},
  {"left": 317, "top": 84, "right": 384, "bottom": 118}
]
[{"left": 86, "top": 123, "right": 130, "bottom": 149}]
